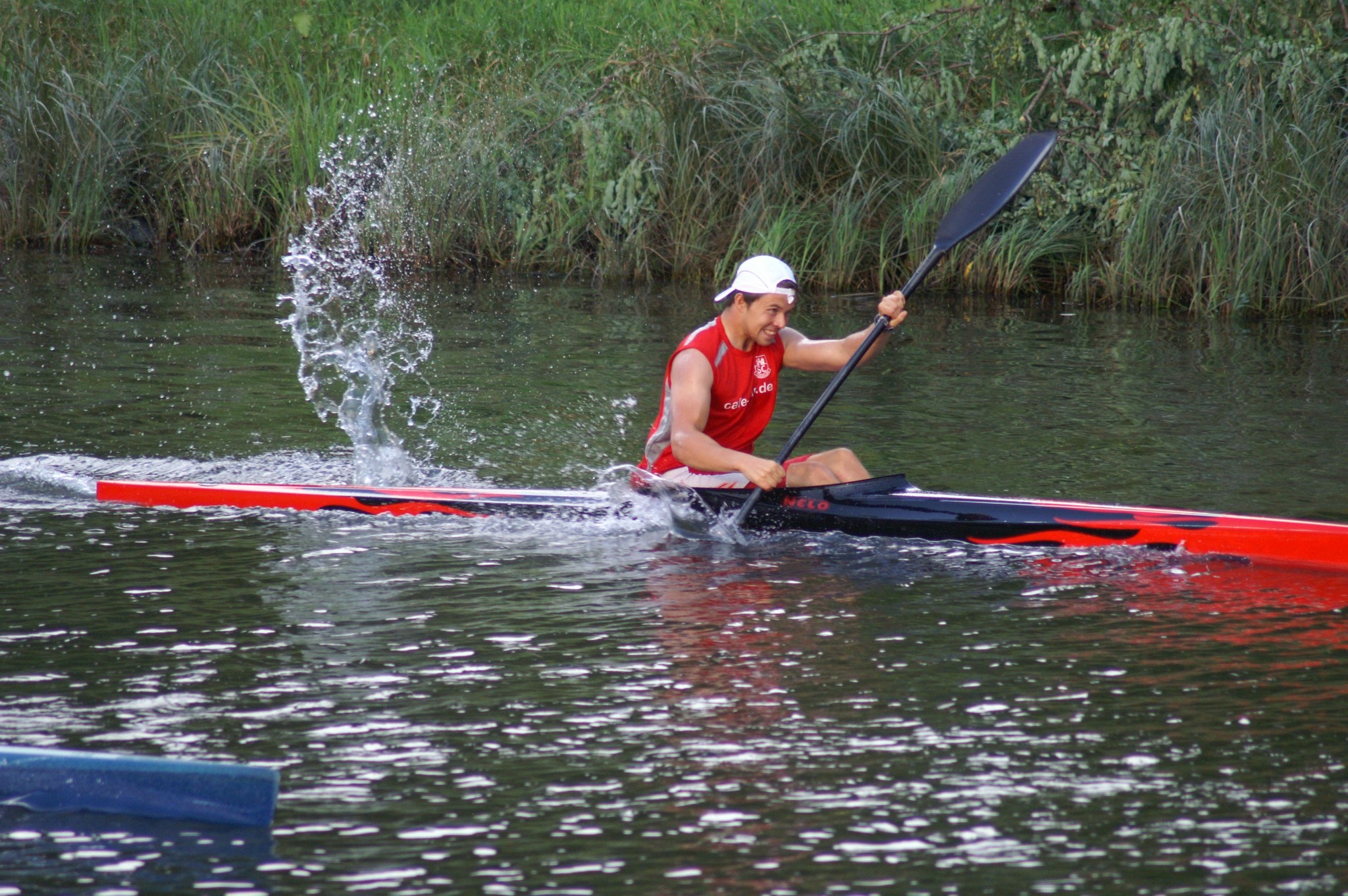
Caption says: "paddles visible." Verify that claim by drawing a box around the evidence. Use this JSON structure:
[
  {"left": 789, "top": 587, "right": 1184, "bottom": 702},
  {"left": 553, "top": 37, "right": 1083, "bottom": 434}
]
[{"left": 732, "top": 130, "right": 1059, "bottom": 525}]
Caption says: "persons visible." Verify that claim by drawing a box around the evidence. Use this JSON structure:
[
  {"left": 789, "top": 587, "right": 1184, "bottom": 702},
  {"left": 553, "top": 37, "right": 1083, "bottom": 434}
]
[
  {"left": 631, "top": 255, "right": 908, "bottom": 489},
  {"left": 647, "top": 539, "right": 800, "bottom": 895}
]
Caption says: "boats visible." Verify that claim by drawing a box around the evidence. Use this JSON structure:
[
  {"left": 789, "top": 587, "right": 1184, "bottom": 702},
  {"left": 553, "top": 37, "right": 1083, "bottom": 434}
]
[
  {"left": 97, "top": 479, "right": 1348, "bottom": 573},
  {"left": 0, "top": 744, "right": 278, "bottom": 825}
]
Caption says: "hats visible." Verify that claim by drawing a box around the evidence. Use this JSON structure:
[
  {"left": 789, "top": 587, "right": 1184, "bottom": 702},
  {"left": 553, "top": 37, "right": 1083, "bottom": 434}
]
[{"left": 714, "top": 255, "right": 797, "bottom": 302}]
